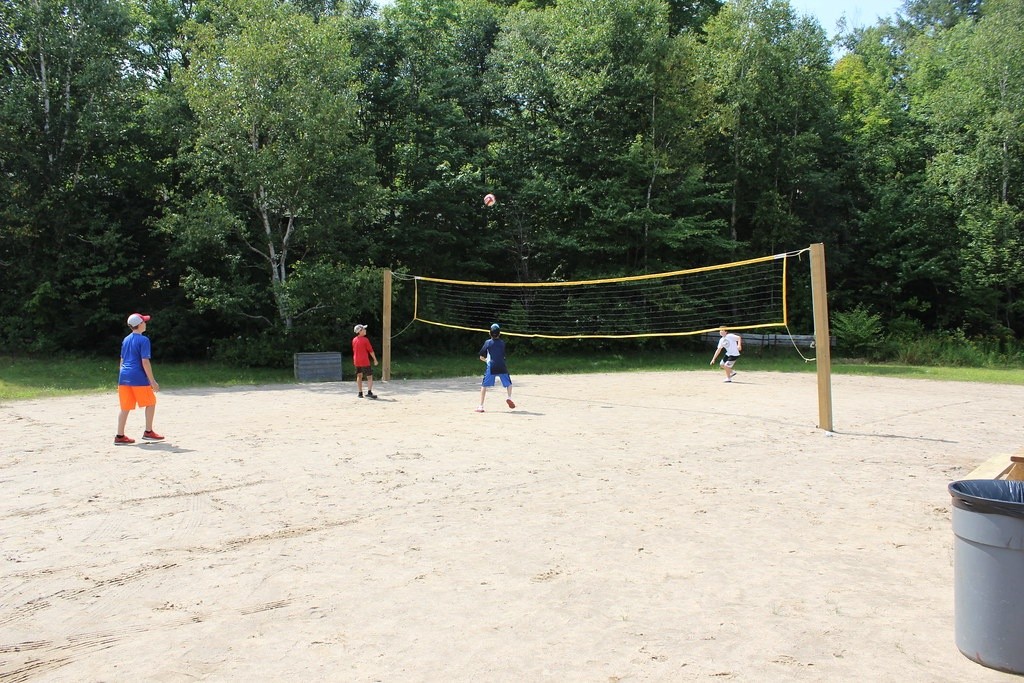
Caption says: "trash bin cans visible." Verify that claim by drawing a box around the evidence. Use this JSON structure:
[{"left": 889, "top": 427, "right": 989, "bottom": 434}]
[{"left": 948, "top": 479, "right": 1023, "bottom": 676}]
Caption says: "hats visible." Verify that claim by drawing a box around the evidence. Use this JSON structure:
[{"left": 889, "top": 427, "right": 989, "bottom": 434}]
[
  {"left": 490, "top": 324, "right": 500, "bottom": 337},
  {"left": 127, "top": 313, "right": 151, "bottom": 327},
  {"left": 354, "top": 324, "right": 368, "bottom": 333}
]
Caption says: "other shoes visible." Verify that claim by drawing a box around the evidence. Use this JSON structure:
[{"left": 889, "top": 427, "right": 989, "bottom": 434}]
[
  {"left": 475, "top": 408, "right": 484, "bottom": 412},
  {"left": 358, "top": 394, "right": 363, "bottom": 398},
  {"left": 368, "top": 391, "right": 373, "bottom": 395},
  {"left": 724, "top": 380, "right": 732, "bottom": 382},
  {"left": 730, "top": 372, "right": 737, "bottom": 377},
  {"left": 506, "top": 399, "right": 515, "bottom": 408}
]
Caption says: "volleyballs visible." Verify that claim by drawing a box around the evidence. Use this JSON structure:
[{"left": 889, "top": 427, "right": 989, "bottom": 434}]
[{"left": 483, "top": 193, "right": 496, "bottom": 206}]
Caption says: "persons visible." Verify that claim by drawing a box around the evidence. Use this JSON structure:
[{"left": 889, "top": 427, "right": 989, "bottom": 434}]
[
  {"left": 710, "top": 326, "right": 742, "bottom": 382},
  {"left": 352, "top": 324, "right": 378, "bottom": 398},
  {"left": 474, "top": 323, "right": 515, "bottom": 412},
  {"left": 114, "top": 314, "right": 165, "bottom": 443}
]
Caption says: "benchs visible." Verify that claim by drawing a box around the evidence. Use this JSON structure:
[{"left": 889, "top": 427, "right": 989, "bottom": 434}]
[
  {"left": 700, "top": 332, "right": 768, "bottom": 360},
  {"left": 769, "top": 334, "right": 836, "bottom": 358}
]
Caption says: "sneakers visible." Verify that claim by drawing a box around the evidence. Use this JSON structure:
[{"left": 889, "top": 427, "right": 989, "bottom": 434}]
[
  {"left": 141, "top": 430, "right": 164, "bottom": 440},
  {"left": 114, "top": 435, "right": 135, "bottom": 444}
]
